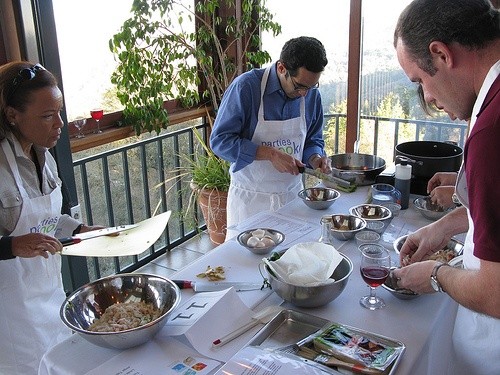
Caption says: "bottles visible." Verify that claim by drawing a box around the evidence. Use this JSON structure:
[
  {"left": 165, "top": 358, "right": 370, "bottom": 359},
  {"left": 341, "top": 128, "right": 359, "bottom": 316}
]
[
  {"left": 395, "top": 159, "right": 412, "bottom": 209},
  {"left": 319, "top": 215, "right": 334, "bottom": 249}
]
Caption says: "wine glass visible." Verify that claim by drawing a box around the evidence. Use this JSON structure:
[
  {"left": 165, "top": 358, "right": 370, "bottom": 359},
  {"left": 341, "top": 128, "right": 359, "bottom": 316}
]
[
  {"left": 90, "top": 106, "right": 104, "bottom": 134},
  {"left": 72, "top": 110, "right": 87, "bottom": 138},
  {"left": 360, "top": 246, "right": 391, "bottom": 310}
]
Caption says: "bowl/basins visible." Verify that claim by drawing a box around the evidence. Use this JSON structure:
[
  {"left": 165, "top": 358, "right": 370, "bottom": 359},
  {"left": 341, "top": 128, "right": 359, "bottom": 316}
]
[
  {"left": 349, "top": 203, "right": 392, "bottom": 235},
  {"left": 355, "top": 231, "right": 380, "bottom": 247},
  {"left": 328, "top": 152, "right": 386, "bottom": 186},
  {"left": 237, "top": 228, "right": 285, "bottom": 255},
  {"left": 358, "top": 243, "right": 384, "bottom": 263},
  {"left": 297, "top": 187, "right": 340, "bottom": 210},
  {"left": 264, "top": 247, "right": 354, "bottom": 308},
  {"left": 382, "top": 269, "right": 422, "bottom": 301},
  {"left": 328, "top": 214, "right": 367, "bottom": 241},
  {"left": 59, "top": 273, "right": 181, "bottom": 349},
  {"left": 394, "top": 233, "right": 464, "bottom": 259},
  {"left": 414, "top": 196, "right": 447, "bottom": 220}
]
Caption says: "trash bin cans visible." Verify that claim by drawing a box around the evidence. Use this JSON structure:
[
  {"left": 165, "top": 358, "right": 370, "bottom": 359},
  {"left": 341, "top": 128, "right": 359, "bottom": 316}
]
[{"left": 393, "top": 140, "right": 464, "bottom": 196}]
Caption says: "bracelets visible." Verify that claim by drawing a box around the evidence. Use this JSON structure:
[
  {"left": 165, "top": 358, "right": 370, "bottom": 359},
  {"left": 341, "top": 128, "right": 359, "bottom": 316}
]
[{"left": 310, "top": 154, "right": 321, "bottom": 161}]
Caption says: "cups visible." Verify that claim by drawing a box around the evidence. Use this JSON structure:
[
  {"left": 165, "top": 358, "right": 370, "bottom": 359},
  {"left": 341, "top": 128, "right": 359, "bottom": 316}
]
[
  {"left": 371, "top": 183, "right": 400, "bottom": 206},
  {"left": 379, "top": 202, "right": 401, "bottom": 243}
]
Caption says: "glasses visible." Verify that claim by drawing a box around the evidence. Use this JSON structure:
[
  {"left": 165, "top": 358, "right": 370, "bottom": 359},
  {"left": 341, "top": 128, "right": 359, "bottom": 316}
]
[
  {"left": 286, "top": 68, "right": 319, "bottom": 91},
  {"left": 5, "top": 63, "right": 46, "bottom": 107}
]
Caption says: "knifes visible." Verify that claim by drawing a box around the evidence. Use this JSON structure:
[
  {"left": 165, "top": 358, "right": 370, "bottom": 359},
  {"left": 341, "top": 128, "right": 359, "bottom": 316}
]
[
  {"left": 169, "top": 279, "right": 266, "bottom": 292},
  {"left": 298, "top": 165, "right": 350, "bottom": 188},
  {"left": 40, "top": 225, "right": 140, "bottom": 251}
]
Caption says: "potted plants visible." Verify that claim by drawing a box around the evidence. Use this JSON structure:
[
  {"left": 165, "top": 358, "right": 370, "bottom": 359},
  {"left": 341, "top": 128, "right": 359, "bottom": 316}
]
[{"left": 109, "top": 0, "right": 282, "bottom": 246}]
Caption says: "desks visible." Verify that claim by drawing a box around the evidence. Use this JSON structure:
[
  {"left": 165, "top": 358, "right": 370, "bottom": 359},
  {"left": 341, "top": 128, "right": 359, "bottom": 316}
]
[{"left": 39, "top": 181, "right": 468, "bottom": 375}]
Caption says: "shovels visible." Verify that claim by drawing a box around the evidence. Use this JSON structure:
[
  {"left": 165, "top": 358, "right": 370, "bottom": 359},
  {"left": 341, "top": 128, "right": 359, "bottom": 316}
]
[{"left": 213, "top": 304, "right": 286, "bottom": 346}]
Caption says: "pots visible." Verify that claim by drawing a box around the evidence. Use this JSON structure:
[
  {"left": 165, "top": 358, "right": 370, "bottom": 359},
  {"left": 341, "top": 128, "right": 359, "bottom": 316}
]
[{"left": 395, "top": 140, "right": 463, "bottom": 195}]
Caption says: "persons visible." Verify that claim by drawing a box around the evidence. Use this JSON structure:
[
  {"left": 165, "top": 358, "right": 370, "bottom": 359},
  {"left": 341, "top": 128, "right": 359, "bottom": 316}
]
[
  {"left": 0, "top": 62, "right": 119, "bottom": 375},
  {"left": 392, "top": 0, "right": 500, "bottom": 375},
  {"left": 210, "top": 37, "right": 333, "bottom": 243}
]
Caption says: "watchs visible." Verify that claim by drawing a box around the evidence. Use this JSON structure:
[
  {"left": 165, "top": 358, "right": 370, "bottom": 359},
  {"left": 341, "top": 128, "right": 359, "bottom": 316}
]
[
  {"left": 429, "top": 261, "right": 452, "bottom": 295},
  {"left": 452, "top": 189, "right": 462, "bottom": 207}
]
